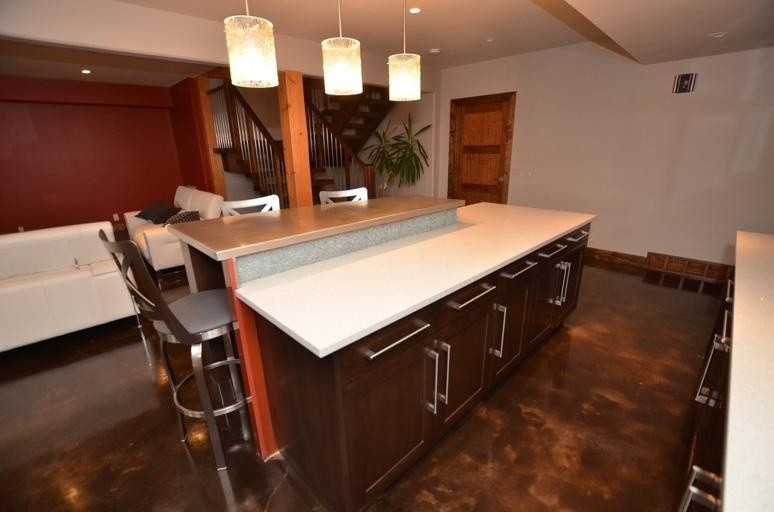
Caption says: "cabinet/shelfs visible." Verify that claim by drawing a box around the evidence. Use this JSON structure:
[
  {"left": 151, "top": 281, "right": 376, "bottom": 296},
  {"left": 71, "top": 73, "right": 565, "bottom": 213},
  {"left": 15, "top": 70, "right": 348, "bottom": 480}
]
[
  {"left": 678, "top": 262, "right": 734, "bottom": 512},
  {"left": 254, "top": 221, "right": 589, "bottom": 512}
]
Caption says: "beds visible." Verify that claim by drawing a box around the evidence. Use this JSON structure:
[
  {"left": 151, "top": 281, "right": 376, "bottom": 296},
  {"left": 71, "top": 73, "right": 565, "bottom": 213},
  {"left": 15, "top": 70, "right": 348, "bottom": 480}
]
[{"left": 0, "top": 220, "right": 142, "bottom": 357}]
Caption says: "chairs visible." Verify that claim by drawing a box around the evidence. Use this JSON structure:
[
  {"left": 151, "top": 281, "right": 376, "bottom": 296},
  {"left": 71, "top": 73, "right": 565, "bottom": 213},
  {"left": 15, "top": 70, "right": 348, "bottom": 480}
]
[
  {"left": 223, "top": 194, "right": 281, "bottom": 216},
  {"left": 95, "top": 229, "right": 254, "bottom": 471},
  {"left": 319, "top": 187, "right": 368, "bottom": 203}
]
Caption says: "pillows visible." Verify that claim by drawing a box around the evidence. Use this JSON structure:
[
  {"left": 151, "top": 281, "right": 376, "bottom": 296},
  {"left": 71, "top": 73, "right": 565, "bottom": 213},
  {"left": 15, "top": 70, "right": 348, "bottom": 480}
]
[
  {"left": 163, "top": 211, "right": 200, "bottom": 226},
  {"left": 135, "top": 205, "right": 183, "bottom": 224}
]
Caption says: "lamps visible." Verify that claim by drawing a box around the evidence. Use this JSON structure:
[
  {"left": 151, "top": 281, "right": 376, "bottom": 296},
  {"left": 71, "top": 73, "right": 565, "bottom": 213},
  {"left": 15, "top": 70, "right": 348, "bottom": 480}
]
[
  {"left": 223, "top": 0, "right": 280, "bottom": 88},
  {"left": 321, "top": 0, "right": 364, "bottom": 96},
  {"left": 389, "top": 0, "right": 422, "bottom": 102}
]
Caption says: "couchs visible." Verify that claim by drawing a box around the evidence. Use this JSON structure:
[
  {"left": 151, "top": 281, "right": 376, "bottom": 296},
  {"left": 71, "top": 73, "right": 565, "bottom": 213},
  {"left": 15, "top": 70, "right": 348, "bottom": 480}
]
[{"left": 124, "top": 186, "right": 224, "bottom": 282}]
